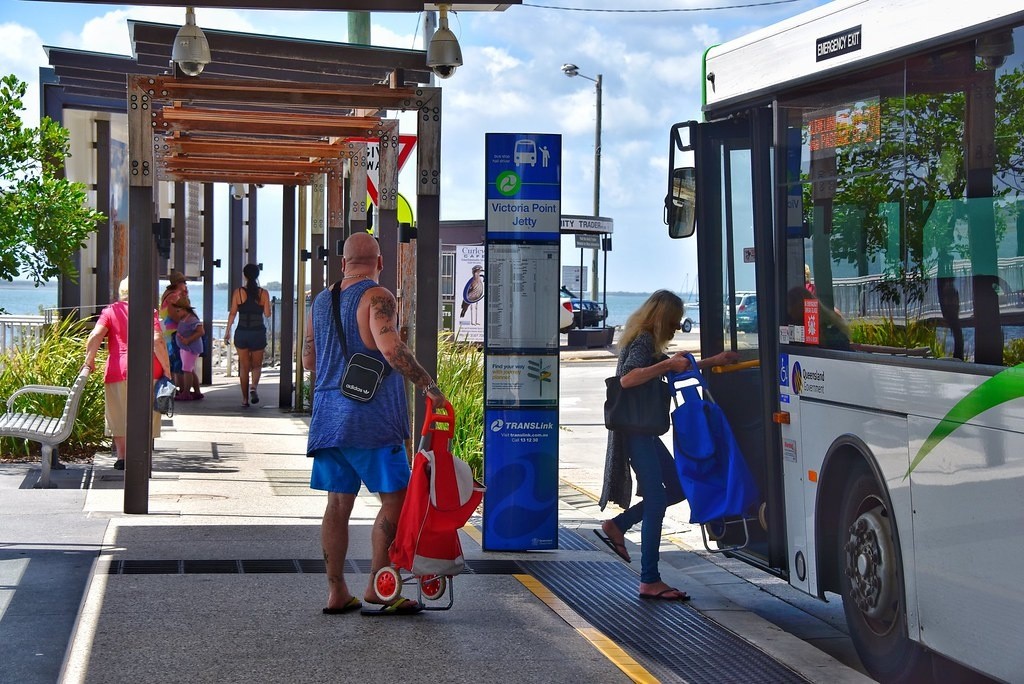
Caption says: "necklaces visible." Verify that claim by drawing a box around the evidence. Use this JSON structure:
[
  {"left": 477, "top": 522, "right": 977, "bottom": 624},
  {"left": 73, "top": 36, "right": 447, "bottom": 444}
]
[{"left": 343, "top": 274, "right": 369, "bottom": 280}]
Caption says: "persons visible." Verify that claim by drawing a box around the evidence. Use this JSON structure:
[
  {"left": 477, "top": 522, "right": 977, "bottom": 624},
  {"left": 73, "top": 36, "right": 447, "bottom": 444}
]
[
  {"left": 79, "top": 276, "right": 171, "bottom": 470},
  {"left": 303, "top": 233, "right": 446, "bottom": 617},
  {"left": 224, "top": 263, "right": 274, "bottom": 408},
  {"left": 161, "top": 272, "right": 188, "bottom": 394},
  {"left": 172, "top": 296, "right": 205, "bottom": 400},
  {"left": 593, "top": 289, "right": 742, "bottom": 602},
  {"left": 787, "top": 286, "right": 853, "bottom": 351}
]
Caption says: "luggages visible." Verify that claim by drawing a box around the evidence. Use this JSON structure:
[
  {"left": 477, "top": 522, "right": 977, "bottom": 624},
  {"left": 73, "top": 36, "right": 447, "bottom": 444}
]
[
  {"left": 373, "top": 396, "right": 487, "bottom": 611},
  {"left": 666, "top": 354, "right": 767, "bottom": 554}
]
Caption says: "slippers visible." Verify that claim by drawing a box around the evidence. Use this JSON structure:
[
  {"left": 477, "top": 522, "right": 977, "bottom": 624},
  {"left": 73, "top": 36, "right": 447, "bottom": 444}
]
[
  {"left": 593, "top": 529, "right": 632, "bottom": 563},
  {"left": 323, "top": 596, "right": 362, "bottom": 614},
  {"left": 638, "top": 587, "right": 690, "bottom": 601},
  {"left": 360, "top": 595, "right": 426, "bottom": 617},
  {"left": 250, "top": 390, "right": 260, "bottom": 404},
  {"left": 240, "top": 402, "right": 249, "bottom": 407}
]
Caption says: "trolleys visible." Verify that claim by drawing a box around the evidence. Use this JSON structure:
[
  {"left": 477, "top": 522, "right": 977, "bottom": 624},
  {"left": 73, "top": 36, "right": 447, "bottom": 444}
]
[{"left": 375, "top": 396, "right": 455, "bottom": 611}]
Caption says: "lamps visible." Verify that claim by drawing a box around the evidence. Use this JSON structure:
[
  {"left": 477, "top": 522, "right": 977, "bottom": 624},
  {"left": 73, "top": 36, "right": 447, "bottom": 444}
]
[
  {"left": 317, "top": 245, "right": 328, "bottom": 259},
  {"left": 152, "top": 217, "right": 171, "bottom": 250},
  {"left": 301, "top": 250, "right": 311, "bottom": 260},
  {"left": 212, "top": 259, "right": 222, "bottom": 268},
  {"left": 335, "top": 239, "right": 344, "bottom": 257},
  {"left": 398, "top": 223, "right": 417, "bottom": 244}
]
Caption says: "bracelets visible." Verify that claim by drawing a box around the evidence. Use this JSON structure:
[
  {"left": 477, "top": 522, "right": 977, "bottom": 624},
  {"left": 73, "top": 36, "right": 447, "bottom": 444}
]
[{"left": 422, "top": 379, "right": 434, "bottom": 396}]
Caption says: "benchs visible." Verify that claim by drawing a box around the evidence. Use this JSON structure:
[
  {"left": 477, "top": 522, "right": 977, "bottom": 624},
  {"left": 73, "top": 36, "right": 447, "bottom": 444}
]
[{"left": 0, "top": 366, "right": 91, "bottom": 489}]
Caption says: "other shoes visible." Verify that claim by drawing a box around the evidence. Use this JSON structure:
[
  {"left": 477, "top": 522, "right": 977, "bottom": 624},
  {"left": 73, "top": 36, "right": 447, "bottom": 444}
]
[
  {"left": 113, "top": 459, "right": 125, "bottom": 470},
  {"left": 192, "top": 392, "right": 204, "bottom": 399},
  {"left": 175, "top": 392, "right": 193, "bottom": 401}
]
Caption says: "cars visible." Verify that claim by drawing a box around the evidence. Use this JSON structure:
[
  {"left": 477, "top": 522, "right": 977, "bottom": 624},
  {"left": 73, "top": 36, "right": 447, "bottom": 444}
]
[
  {"left": 723, "top": 291, "right": 758, "bottom": 334},
  {"left": 559, "top": 289, "right": 603, "bottom": 329}
]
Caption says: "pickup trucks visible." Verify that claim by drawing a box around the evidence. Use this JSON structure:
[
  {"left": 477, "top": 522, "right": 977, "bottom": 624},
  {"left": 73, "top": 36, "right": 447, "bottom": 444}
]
[{"left": 680, "top": 302, "right": 701, "bottom": 334}]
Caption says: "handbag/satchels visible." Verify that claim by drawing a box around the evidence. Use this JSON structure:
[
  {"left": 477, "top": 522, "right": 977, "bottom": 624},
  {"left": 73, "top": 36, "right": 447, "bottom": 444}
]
[
  {"left": 152, "top": 376, "right": 176, "bottom": 418},
  {"left": 604, "top": 343, "right": 670, "bottom": 436},
  {"left": 340, "top": 352, "right": 384, "bottom": 403}
]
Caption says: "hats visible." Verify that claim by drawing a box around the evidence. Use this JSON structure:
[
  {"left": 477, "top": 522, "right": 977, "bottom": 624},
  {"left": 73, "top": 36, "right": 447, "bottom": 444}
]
[
  {"left": 170, "top": 296, "right": 196, "bottom": 309},
  {"left": 166, "top": 272, "right": 189, "bottom": 289}
]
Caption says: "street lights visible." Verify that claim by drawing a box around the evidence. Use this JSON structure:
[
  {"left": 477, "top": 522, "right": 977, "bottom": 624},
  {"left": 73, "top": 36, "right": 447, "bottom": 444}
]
[{"left": 562, "top": 62, "right": 601, "bottom": 326}]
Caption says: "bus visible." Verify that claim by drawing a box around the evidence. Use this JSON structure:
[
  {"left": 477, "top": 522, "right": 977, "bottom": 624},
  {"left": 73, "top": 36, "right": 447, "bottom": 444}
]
[{"left": 663, "top": 1, "right": 1024, "bottom": 684}]
